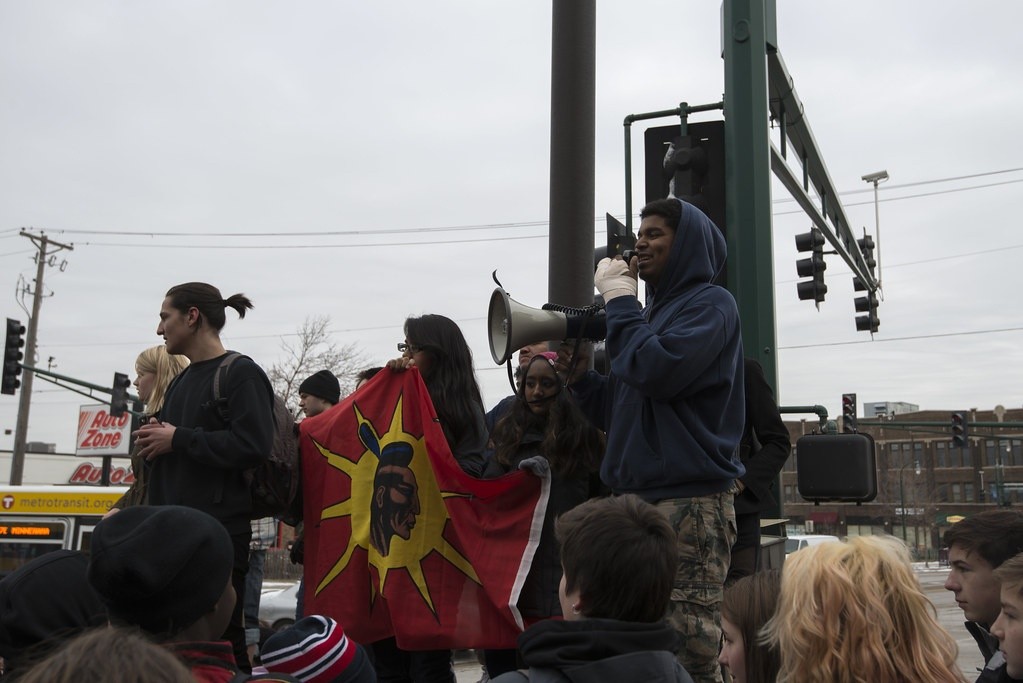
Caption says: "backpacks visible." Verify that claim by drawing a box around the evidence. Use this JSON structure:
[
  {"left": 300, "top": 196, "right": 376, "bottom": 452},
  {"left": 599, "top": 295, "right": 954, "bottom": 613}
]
[{"left": 210, "top": 352, "right": 301, "bottom": 507}]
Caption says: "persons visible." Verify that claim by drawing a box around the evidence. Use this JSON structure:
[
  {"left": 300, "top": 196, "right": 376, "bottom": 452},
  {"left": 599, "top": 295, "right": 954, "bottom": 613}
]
[
  {"left": 0, "top": 549, "right": 111, "bottom": 683},
  {"left": 14, "top": 628, "right": 192, "bottom": 683},
  {"left": 485, "top": 341, "right": 561, "bottom": 438},
  {"left": 484, "top": 350, "right": 611, "bottom": 678},
  {"left": 720, "top": 569, "right": 782, "bottom": 683},
  {"left": 556, "top": 199, "right": 747, "bottom": 683},
  {"left": 728, "top": 357, "right": 791, "bottom": 585},
  {"left": 355, "top": 365, "right": 380, "bottom": 390},
  {"left": 373, "top": 313, "right": 489, "bottom": 683},
  {"left": 757, "top": 533, "right": 967, "bottom": 683},
  {"left": 490, "top": 494, "right": 694, "bottom": 683},
  {"left": 989, "top": 552, "right": 1023, "bottom": 679},
  {"left": 87, "top": 506, "right": 251, "bottom": 682},
  {"left": 943, "top": 508, "right": 1023, "bottom": 683},
  {"left": 101, "top": 345, "right": 188, "bottom": 521},
  {"left": 257, "top": 613, "right": 376, "bottom": 682},
  {"left": 130, "top": 282, "right": 275, "bottom": 676},
  {"left": 289, "top": 370, "right": 341, "bottom": 624}
]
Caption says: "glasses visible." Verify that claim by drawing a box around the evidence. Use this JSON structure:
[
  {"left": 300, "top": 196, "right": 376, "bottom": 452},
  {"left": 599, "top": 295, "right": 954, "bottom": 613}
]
[{"left": 397, "top": 343, "right": 428, "bottom": 352}]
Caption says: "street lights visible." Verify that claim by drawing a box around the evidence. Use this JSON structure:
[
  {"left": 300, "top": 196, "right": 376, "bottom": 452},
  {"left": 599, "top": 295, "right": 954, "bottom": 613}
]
[{"left": 900, "top": 458, "right": 922, "bottom": 541}]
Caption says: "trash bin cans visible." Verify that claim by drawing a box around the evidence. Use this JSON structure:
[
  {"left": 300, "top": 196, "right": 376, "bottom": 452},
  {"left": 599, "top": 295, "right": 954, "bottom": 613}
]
[{"left": 939, "top": 550, "right": 948, "bottom": 566}]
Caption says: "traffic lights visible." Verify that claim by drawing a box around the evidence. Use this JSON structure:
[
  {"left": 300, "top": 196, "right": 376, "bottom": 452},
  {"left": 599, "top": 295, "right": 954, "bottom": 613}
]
[
  {"left": 795, "top": 228, "right": 828, "bottom": 301},
  {"left": 111, "top": 372, "right": 131, "bottom": 417},
  {"left": 0, "top": 317, "right": 26, "bottom": 395},
  {"left": 852, "top": 234, "right": 880, "bottom": 333},
  {"left": 842, "top": 393, "right": 857, "bottom": 435},
  {"left": 952, "top": 410, "right": 968, "bottom": 445}
]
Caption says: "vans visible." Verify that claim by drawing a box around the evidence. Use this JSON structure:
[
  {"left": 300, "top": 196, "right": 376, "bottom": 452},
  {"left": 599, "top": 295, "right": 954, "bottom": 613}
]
[{"left": 786, "top": 534, "right": 840, "bottom": 560}]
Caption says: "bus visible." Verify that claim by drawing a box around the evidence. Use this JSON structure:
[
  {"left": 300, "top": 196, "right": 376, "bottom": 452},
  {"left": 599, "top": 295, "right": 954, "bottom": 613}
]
[
  {"left": 0, "top": 450, "right": 136, "bottom": 487},
  {"left": 0, "top": 485, "right": 131, "bottom": 581}
]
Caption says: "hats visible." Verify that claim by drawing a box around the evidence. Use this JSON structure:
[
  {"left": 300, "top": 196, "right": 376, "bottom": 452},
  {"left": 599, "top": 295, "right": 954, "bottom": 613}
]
[
  {"left": 530, "top": 350, "right": 575, "bottom": 389},
  {"left": 88, "top": 505, "right": 236, "bottom": 642},
  {"left": 261, "top": 615, "right": 375, "bottom": 683},
  {"left": 298, "top": 370, "right": 341, "bottom": 404}
]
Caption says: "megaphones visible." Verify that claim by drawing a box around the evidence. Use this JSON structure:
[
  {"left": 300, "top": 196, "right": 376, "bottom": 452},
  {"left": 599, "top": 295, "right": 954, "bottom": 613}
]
[{"left": 488, "top": 286, "right": 608, "bottom": 365}]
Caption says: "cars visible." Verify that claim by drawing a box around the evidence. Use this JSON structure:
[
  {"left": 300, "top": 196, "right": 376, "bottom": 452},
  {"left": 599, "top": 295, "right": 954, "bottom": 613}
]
[{"left": 258, "top": 581, "right": 302, "bottom": 633}]
